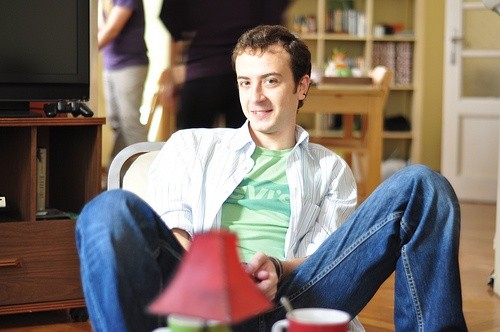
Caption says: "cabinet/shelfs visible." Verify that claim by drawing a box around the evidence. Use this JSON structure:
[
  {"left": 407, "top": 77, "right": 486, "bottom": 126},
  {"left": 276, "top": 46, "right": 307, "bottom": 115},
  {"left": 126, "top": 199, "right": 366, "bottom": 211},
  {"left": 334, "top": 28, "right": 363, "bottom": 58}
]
[
  {"left": 285, "top": 0, "right": 444, "bottom": 172},
  {"left": 0, "top": 117, "right": 107, "bottom": 322}
]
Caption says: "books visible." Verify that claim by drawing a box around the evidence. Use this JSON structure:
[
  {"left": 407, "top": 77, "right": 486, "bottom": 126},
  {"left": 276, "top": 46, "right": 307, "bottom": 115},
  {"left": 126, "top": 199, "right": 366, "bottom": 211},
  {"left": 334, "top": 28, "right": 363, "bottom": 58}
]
[
  {"left": 334, "top": 10, "right": 364, "bottom": 34},
  {"left": 36, "top": 147, "right": 50, "bottom": 214}
]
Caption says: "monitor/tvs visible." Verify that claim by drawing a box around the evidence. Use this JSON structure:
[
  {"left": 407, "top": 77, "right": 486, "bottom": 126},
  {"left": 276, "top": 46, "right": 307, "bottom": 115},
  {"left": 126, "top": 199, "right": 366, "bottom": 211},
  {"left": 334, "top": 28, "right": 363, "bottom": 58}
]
[{"left": 0, "top": 0, "right": 90, "bottom": 117}]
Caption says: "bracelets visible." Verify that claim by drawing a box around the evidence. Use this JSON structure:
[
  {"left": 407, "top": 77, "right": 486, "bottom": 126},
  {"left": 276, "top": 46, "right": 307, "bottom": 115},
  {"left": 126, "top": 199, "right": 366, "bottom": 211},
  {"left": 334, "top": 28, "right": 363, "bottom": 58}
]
[{"left": 269, "top": 256, "right": 284, "bottom": 279}]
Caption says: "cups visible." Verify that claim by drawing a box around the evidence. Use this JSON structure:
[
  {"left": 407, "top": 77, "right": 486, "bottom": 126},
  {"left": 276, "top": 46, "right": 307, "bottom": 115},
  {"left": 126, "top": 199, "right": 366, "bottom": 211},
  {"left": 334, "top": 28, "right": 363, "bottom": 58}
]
[{"left": 271, "top": 307, "right": 351, "bottom": 332}]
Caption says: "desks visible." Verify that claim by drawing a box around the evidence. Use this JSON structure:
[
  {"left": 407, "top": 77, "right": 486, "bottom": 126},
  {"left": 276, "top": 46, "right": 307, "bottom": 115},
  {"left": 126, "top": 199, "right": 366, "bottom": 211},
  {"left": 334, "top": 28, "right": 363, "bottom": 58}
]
[{"left": 297, "top": 84, "right": 386, "bottom": 199}]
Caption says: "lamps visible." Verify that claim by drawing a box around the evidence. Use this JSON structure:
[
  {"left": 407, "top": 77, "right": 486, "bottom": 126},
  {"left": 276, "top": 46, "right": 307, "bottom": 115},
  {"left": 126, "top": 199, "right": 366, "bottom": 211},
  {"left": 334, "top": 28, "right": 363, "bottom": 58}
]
[{"left": 148, "top": 230, "right": 272, "bottom": 332}]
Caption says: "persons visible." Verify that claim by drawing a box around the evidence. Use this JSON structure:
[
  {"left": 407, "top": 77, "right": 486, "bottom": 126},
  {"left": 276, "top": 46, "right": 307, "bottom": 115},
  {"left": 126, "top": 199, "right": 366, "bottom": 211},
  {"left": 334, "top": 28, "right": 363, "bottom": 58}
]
[
  {"left": 74, "top": 24, "right": 468, "bottom": 332},
  {"left": 97, "top": 0, "right": 150, "bottom": 163},
  {"left": 160, "top": 0, "right": 290, "bottom": 129}
]
